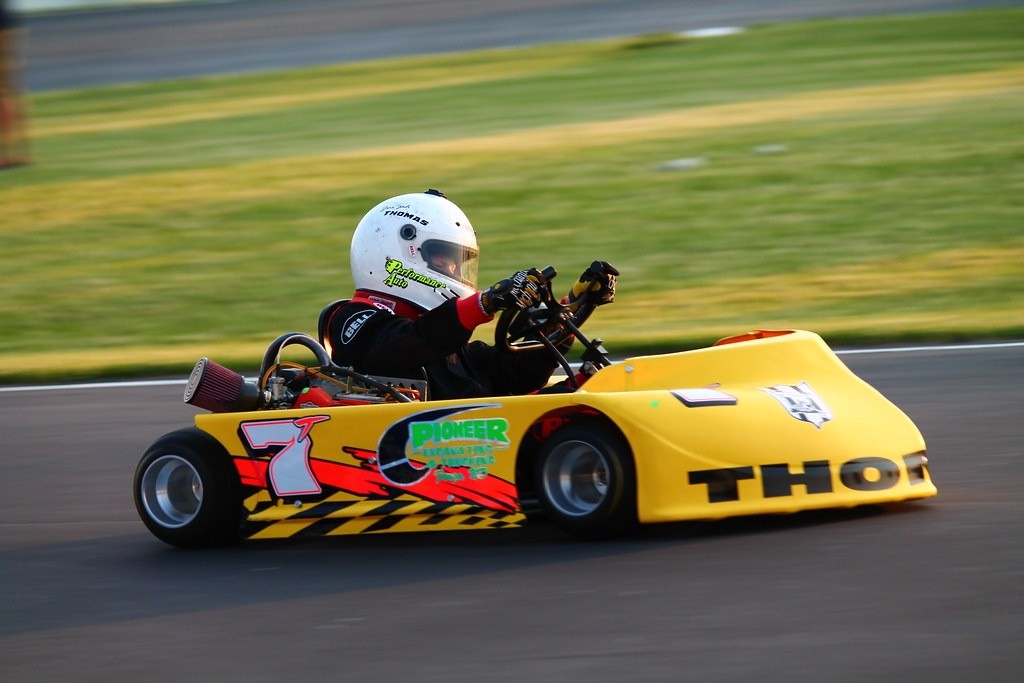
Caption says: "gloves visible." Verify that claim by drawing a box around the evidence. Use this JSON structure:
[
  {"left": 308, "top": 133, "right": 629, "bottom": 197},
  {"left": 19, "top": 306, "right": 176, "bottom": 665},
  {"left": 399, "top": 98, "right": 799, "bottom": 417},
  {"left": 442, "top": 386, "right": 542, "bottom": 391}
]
[
  {"left": 481, "top": 267, "right": 551, "bottom": 313},
  {"left": 569, "top": 262, "right": 620, "bottom": 306}
]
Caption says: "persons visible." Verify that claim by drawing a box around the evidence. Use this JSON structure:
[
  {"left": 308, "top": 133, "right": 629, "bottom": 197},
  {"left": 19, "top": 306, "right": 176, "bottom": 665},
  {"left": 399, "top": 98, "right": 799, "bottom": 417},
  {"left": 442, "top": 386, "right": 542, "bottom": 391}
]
[{"left": 316, "top": 191, "right": 617, "bottom": 399}]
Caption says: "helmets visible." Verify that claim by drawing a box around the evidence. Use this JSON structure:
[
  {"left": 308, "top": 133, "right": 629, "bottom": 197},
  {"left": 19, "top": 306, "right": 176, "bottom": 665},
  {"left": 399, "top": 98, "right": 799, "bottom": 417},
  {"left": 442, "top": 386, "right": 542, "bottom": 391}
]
[{"left": 350, "top": 192, "right": 481, "bottom": 310}]
